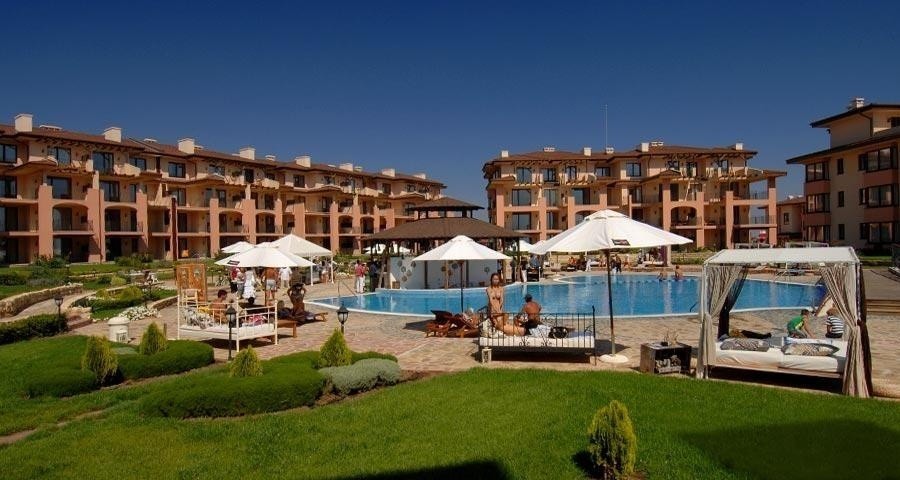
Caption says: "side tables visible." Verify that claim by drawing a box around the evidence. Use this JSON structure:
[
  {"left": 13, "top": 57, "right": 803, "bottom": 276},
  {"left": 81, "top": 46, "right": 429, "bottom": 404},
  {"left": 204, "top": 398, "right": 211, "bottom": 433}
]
[{"left": 640, "top": 341, "right": 692, "bottom": 374}]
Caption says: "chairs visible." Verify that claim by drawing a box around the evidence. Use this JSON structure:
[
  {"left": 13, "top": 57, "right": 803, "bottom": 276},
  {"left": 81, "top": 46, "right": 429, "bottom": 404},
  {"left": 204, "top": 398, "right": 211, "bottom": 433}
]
[
  {"left": 239, "top": 302, "right": 328, "bottom": 338},
  {"left": 426, "top": 309, "right": 479, "bottom": 338}
]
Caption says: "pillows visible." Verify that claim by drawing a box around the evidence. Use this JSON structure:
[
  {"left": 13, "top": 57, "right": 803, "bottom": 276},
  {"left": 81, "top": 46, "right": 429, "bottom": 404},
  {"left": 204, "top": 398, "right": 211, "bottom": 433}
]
[
  {"left": 781, "top": 344, "right": 839, "bottom": 356},
  {"left": 480, "top": 319, "right": 491, "bottom": 336},
  {"left": 721, "top": 338, "right": 769, "bottom": 352},
  {"left": 182, "top": 307, "right": 199, "bottom": 326},
  {"left": 191, "top": 313, "right": 214, "bottom": 329},
  {"left": 489, "top": 326, "right": 506, "bottom": 338}
]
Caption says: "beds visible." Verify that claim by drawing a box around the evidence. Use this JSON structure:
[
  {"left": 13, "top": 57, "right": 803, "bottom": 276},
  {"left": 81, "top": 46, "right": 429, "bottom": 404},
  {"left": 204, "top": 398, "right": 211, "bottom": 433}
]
[
  {"left": 477, "top": 305, "right": 596, "bottom": 365},
  {"left": 698, "top": 245, "right": 874, "bottom": 399},
  {"left": 177, "top": 291, "right": 278, "bottom": 352}
]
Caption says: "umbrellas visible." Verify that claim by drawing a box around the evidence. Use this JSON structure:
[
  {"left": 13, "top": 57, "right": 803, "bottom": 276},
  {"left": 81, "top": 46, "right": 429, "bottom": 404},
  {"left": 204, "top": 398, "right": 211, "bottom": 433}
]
[
  {"left": 218, "top": 232, "right": 333, "bottom": 257},
  {"left": 362, "top": 240, "right": 411, "bottom": 256},
  {"left": 213, "top": 241, "right": 317, "bottom": 307},
  {"left": 504, "top": 241, "right": 531, "bottom": 264},
  {"left": 526, "top": 208, "right": 694, "bottom": 355},
  {"left": 411, "top": 234, "right": 512, "bottom": 314},
  {"left": 528, "top": 240, "right": 549, "bottom": 255}
]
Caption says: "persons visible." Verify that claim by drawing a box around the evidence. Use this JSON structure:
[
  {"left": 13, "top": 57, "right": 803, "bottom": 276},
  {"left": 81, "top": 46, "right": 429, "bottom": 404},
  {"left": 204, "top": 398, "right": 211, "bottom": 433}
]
[
  {"left": 498, "top": 247, "right": 661, "bottom": 285},
  {"left": 513, "top": 293, "right": 542, "bottom": 328},
  {"left": 142, "top": 271, "right": 165, "bottom": 293},
  {"left": 486, "top": 273, "right": 504, "bottom": 318},
  {"left": 206, "top": 256, "right": 389, "bottom": 323},
  {"left": 824, "top": 308, "right": 844, "bottom": 338},
  {"left": 672, "top": 264, "right": 683, "bottom": 280},
  {"left": 490, "top": 312, "right": 576, "bottom": 338},
  {"left": 656, "top": 267, "right": 668, "bottom": 282},
  {"left": 786, "top": 309, "right": 817, "bottom": 339},
  {"left": 456, "top": 307, "right": 487, "bottom": 328}
]
